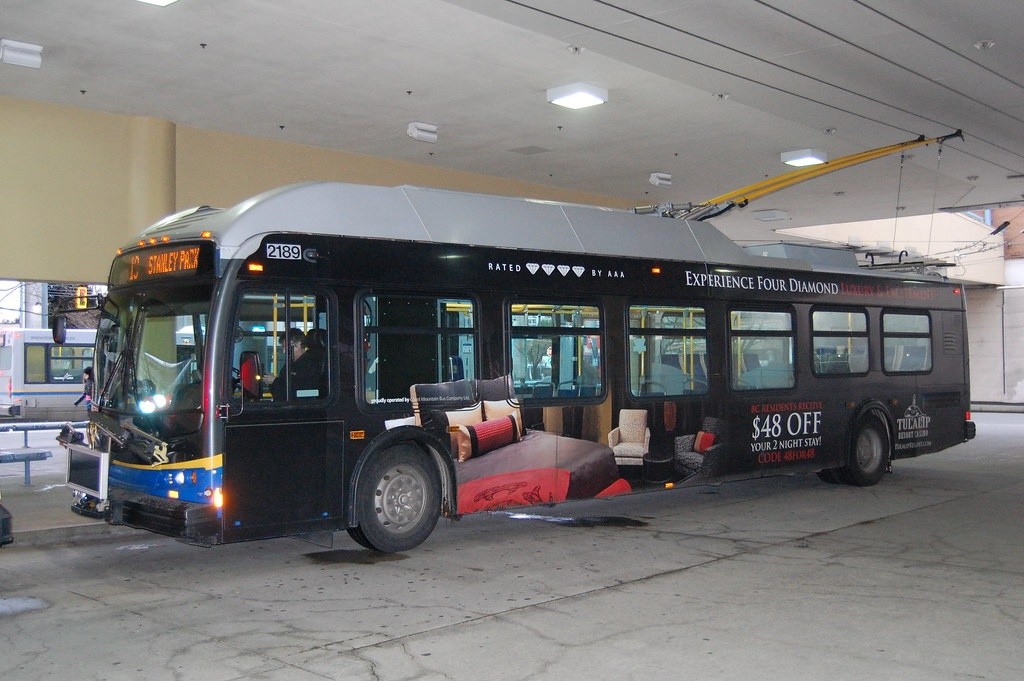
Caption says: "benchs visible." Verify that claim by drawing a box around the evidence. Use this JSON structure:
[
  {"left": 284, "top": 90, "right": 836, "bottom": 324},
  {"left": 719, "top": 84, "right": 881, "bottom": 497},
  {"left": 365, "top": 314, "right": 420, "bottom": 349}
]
[{"left": 0, "top": 448, "right": 52, "bottom": 487}]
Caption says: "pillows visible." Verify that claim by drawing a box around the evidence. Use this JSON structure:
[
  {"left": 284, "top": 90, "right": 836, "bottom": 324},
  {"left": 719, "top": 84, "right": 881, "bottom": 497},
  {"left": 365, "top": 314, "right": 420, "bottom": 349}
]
[
  {"left": 456, "top": 411, "right": 524, "bottom": 463},
  {"left": 483, "top": 399, "right": 523, "bottom": 436},
  {"left": 445, "top": 400, "right": 483, "bottom": 460},
  {"left": 693, "top": 431, "right": 716, "bottom": 454}
]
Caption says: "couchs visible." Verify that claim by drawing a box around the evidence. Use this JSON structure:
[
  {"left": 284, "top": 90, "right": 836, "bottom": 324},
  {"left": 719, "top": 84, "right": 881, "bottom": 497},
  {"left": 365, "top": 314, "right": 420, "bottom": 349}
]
[
  {"left": 674, "top": 416, "right": 724, "bottom": 478},
  {"left": 608, "top": 408, "right": 650, "bottom": 465}
]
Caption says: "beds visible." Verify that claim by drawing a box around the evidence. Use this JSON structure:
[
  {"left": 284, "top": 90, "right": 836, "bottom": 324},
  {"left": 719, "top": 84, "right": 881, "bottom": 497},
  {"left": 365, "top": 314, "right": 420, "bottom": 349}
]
[{"left": 410, "top": 377, "right": 620, "bottom": 514}]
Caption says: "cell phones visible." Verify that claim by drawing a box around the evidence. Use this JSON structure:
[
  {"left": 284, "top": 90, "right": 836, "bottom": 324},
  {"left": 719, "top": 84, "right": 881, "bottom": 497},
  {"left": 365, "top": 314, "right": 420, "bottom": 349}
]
[{"left": 262, "top": 372, "right": 267, "bottom": 377}]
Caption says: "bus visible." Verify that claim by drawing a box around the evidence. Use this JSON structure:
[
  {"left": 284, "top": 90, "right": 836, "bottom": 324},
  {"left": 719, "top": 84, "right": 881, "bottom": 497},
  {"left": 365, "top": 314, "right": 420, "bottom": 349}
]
[
  {"left": 0, "top": 324, "right": 206, "bottom": 422},
  {"left": 69, "top": 182, "right": 976, "bottom": 554}
]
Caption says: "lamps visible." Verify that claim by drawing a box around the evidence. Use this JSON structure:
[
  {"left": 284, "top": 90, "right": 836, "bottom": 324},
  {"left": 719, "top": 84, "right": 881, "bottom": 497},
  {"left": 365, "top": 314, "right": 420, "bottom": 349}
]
[
  {"left": 407, "top": 121, "right": 438, "bottom": 142},
  {"left": 546, "top": 82, "right": 608, "bottom": 110},
  {"left": 649, "top": 173, "right": 672, "bottom": 189},
  {"left": 781, "top": 148, "right": 827, "bottom": 167},
  {"left": 0, "top": 38, "right": 43, "bottom": 68}
]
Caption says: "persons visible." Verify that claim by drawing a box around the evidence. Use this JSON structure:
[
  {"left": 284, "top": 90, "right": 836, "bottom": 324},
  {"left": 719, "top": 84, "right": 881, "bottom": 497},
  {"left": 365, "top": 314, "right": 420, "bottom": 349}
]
[
  {"left": 263, "top": 328, "right": 322, "bottom": 401},
  {"left": 74, "top": 366, "right": 93, "bottom": 417}
]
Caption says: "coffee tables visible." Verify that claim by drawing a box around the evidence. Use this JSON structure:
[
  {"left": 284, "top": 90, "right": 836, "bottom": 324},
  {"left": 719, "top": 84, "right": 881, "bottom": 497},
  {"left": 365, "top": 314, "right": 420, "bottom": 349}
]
[{"left": 643, "top": 452, "right": 674, "bottom": 484}]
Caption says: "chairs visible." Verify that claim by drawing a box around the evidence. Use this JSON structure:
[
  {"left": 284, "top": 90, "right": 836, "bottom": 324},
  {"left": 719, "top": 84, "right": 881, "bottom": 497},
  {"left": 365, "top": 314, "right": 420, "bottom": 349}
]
[
  {"left": 514, "top": 380, "right": 749, "bottom": 400},
  {"left": 306, "top": 329, "right": 328, "bottom": 374}
]
